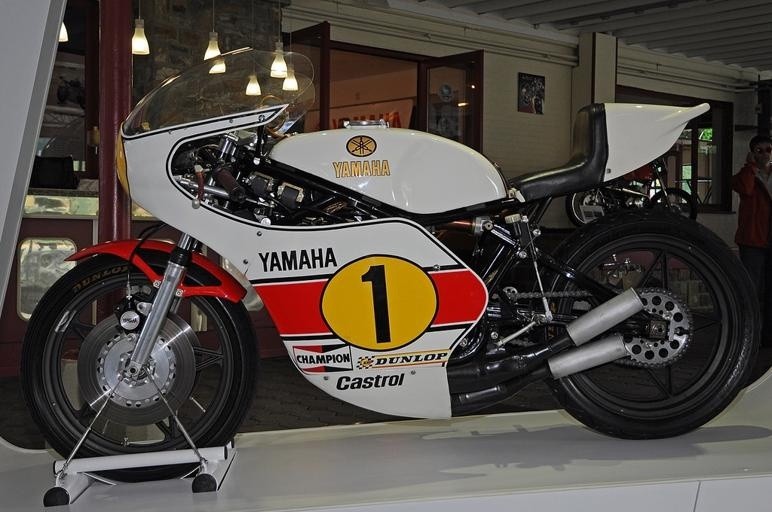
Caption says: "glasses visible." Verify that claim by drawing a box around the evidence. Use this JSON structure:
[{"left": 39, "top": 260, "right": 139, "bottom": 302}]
[{"left": 754, "top": 147, "right": 772, "bottom": 153}]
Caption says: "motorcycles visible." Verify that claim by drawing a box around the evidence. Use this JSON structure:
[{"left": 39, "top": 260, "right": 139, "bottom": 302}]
[
  {"left": 564, "top": 151, "right": 698, "bottom": 226},
  {"left": 21, "top": 41, "right": 760, "bottom": 483}
]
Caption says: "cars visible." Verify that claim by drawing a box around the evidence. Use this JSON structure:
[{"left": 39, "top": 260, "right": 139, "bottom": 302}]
[{"left": 55, "top": 74, "right": 85, "bottom": 110}]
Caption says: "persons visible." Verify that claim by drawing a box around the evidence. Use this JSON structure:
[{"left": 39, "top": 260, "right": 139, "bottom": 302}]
[{"left": 728, "top": 133, "right": 771, "bottom": 349}]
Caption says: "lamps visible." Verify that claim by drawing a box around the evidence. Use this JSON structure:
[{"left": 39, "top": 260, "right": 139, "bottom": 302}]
[
  {"left": 204, "top": 0, "right": 299, "bottom": 97},
  {"left": 130, "top": 1, "right": 150, "bottom": 56}
]
